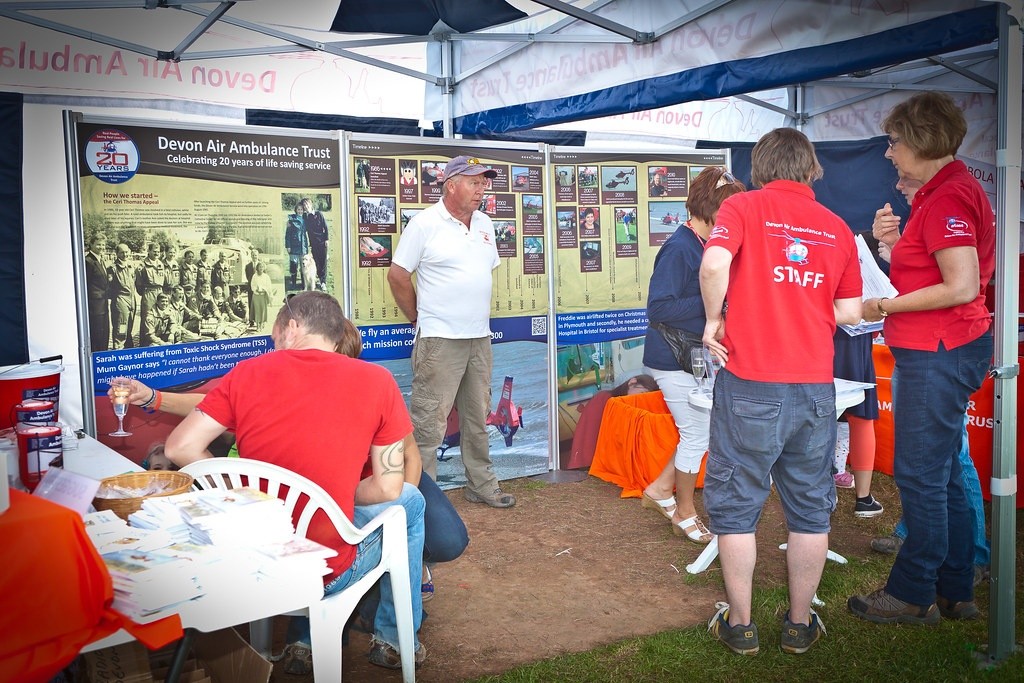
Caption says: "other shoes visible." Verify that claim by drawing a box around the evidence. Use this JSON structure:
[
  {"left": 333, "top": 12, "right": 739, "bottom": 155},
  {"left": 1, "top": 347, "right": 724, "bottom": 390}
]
[
  {"left": 284, "top": 644, "right": 312, "bottom": 675},
  {"left": 368, "top": 639, "right": 427, "bottom": 669}
]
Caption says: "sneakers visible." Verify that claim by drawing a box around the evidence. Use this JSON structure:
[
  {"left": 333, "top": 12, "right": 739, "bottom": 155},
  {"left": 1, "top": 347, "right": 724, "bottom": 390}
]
[
  {"left": 705, "top": 601, "right": 759, "bottom": 654},
  {"left": 871, "top": 536, "right": 904, "bottom": 552},
  {"left": 853, "top": 498, "right": 884, "bottom": 519},
  {"left": 835, "top": 471, "right": 855, "bottom": 489},
  {"left": 848, "top": 588, "right": 941, "bottom": 623},
  {"left": 464, "top": 487, "right": 516, "bottom": 508},
  {"left": 937, "top": 594, "right": 980, "bottom": 620},
  {"left": 781, "top": 607, "right": 822, "bottom": 654}
]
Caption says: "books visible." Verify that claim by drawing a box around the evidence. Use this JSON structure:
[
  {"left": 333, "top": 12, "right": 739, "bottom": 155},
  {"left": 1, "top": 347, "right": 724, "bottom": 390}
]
[{"left": 81, "top": 485, "right": 339, "bottom": 627}]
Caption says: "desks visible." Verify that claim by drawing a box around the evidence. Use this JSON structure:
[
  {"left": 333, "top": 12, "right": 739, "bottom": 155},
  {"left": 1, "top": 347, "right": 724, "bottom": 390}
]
[
  {"left": 684, "top": 375, "right": 880, "bottom": 607},
  {"left": 0, "top": 428, "right": 341, "bottom": 683}
]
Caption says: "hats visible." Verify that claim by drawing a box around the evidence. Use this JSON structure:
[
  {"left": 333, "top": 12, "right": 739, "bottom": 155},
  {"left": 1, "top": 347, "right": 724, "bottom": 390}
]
[{"left": 443, "top": 154, "right": 497, "bottom": 184}]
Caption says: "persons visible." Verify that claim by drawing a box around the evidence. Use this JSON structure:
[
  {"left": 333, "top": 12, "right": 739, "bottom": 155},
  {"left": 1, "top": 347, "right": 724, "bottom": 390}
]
[
  {"left": 580, "top": 165, "right": 666, "bottom": 256},
  {"left": 848, "top": 88, "right": 993, "bottom": 625},
  {"left": 832, "top": 232, "right": 889, "bottom": 518},
  {"left": 245, "top": 250, "right": 272, "bottom": 334},
  {"left": 641, "top": 165, "right": 748, "bottom": 543},
  {"left": 386, "top": 154, "right": 516, "bottom": 509},
  {"left": 86, "top": 234, "right": 249, "bottom": 352},
  {"left": 568, "top": 373, "right": 659, "bottom": 469},
  {"left": 286, "top": 199, "right": 329, "bottom": 292},
  {"left": 400, "top": 163, "right": 443, "bottom": 187},
  {"left": 357, "top": 160, "right": 391, "bottom": 224},
  {"left": 142, "top": 438, "right": 178, "bottom": 471},
  {"left": 106, "top": 291, "right": 469, "bottom": 672},
  {"left": 699, "top": 128, "right": 863, "bottom": 656},
  {"left": 498, "top": 224, "right": 512, "bottom": 242}
]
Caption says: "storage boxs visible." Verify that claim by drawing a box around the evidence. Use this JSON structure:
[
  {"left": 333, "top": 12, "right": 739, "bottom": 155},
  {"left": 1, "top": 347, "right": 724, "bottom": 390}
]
[{"left": 82, "top": 614, "right": 273, "bottom": 683}]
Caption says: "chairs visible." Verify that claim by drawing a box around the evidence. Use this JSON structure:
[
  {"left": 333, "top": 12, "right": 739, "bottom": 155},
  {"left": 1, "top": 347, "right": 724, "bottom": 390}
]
[{"left": 166, "top": 456, "right": 416, "bottom": 683}]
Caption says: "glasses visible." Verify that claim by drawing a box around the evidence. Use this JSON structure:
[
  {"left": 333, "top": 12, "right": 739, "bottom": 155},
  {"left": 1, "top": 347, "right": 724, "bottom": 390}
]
[
  {"left": 716, "top": 172, "right": 735, "bottom": 190},
  {"left": 889, "top": 137, "right": 904, "bottom": 148}
]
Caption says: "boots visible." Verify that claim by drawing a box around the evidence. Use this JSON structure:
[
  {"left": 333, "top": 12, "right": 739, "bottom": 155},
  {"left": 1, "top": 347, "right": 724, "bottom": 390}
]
[
  {"left": 301, "top": 274, "right": 305, "bottom": 290},
  {"left": 290, "top": 273, "right": 297, "bottom": 290}
]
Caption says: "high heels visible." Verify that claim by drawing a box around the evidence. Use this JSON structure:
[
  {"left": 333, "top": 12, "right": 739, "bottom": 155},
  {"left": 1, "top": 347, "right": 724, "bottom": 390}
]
[
  {"left": 670, "top": 512, "right": 714, "bottom": 544},
  {"left": 642, "top": 491, "right": 678, "bottom": 519},
  {"left": 321, "top": 282, "right": 326, "bottom": 290}
]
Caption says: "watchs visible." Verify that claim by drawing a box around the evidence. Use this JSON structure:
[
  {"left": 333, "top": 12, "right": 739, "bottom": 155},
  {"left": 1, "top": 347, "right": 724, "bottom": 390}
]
[{"left": 878, "top": 297, "right": 888, "bottom": 318}]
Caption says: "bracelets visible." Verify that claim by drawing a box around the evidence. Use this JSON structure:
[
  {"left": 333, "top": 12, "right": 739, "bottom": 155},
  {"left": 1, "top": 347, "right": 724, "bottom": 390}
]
[{"left": 136, "top": 388, "right": 162, "bottom": 414}]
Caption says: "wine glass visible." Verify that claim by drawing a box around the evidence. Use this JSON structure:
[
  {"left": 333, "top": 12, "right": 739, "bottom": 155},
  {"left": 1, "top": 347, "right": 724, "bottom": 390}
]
[
  {"left": 691, "top": 348, "right": 716, "bottom": 400},
  {"left": 109, "top": 378, "right": 132, "bottom": 437}
]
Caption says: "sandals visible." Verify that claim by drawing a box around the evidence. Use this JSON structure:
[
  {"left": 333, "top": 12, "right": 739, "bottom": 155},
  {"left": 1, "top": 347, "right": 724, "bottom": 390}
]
[{"left": 419, "top": 562, "right": 433, "bottom": 602}]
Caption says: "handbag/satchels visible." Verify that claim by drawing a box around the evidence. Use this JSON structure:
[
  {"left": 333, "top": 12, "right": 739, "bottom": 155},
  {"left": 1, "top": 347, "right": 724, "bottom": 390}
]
[{"left": 648, "top": 320, "right": 704, "bottom": 375}]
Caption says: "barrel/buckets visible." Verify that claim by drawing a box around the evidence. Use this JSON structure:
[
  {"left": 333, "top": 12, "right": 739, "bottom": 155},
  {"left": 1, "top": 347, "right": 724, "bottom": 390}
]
[{"left": 1, "top": 355, "right": 64, "bottom": 438}]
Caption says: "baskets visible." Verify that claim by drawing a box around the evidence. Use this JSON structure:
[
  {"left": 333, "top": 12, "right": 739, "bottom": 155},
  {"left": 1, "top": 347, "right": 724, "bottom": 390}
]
[{"left": 92, "top": 471, "right": 194, "bottom": 519}]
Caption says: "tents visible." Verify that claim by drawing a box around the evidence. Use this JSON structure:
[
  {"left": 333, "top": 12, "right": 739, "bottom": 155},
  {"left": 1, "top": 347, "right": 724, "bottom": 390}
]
[{"left": 0, "top": 0, "right": 1024, "bottom": 660}]
